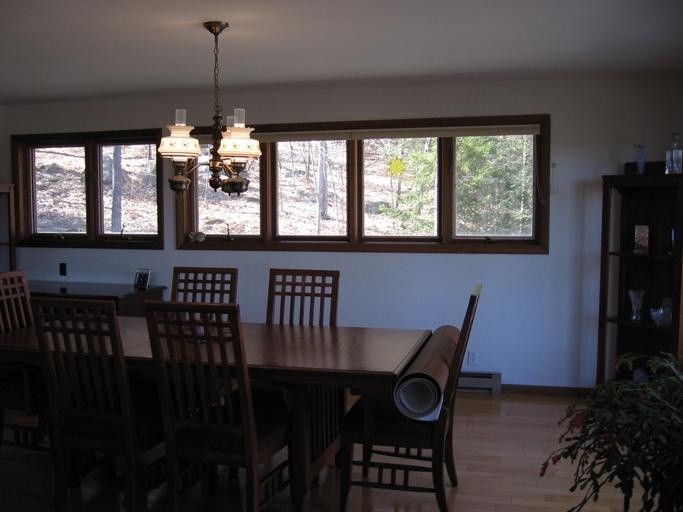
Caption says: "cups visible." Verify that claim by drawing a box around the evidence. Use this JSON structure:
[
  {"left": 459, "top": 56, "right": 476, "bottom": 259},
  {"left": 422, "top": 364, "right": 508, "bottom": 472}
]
[{"left": 634, "top": 142, "right": 646, "bottom": 174}]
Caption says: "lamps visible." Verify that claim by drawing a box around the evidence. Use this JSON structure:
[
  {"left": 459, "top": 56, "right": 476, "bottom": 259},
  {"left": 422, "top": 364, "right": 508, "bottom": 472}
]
[{"left": 157, "top": 21, "right": 263, "bottom": 197}]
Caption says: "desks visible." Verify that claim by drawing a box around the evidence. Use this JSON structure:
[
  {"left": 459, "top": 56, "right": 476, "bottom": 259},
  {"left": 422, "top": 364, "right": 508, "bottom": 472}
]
[
  {"left": 28, "top": 279, "right": 165, "bottom": 315},
  {"left": 3, "top": 316, "right": 432, "bottom": 512}
]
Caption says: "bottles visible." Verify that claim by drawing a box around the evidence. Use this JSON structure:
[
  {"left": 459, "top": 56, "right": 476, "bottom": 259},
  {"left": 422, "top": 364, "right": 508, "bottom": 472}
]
[{"left": 664, "top": 133, "right": 683, "bottom": 174}]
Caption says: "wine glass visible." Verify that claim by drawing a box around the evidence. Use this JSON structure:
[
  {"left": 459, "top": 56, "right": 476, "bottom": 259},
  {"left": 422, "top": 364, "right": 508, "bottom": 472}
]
[{"left": 627, "top": 289, "right": 646, "bottom": 321}]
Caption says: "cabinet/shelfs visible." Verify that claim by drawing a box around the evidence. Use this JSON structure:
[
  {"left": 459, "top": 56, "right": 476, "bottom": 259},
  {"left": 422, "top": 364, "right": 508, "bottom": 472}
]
[{"left": 594, "top": 162, "right": 683, "bottom": 392}]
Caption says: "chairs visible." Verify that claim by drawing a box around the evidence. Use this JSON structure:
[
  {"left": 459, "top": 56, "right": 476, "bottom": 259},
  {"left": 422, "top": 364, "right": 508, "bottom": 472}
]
[
  {"left": 142, "top": 302, "right": 293, "bottom": 510},
  {"left": 29, "top": 296, "right": 138, "bottom": 511},
  {"left": 0, "top": 270, "right": 35, "bottom": 334},
  {"left": 172, "top": 267, "right": 239, "bottom": 322},
  {"left": 267, "top": 269, "right": 340, "bottom": 327},
  {"left": 340, "top": 284, "right": 479, "bottom": 511}
]
